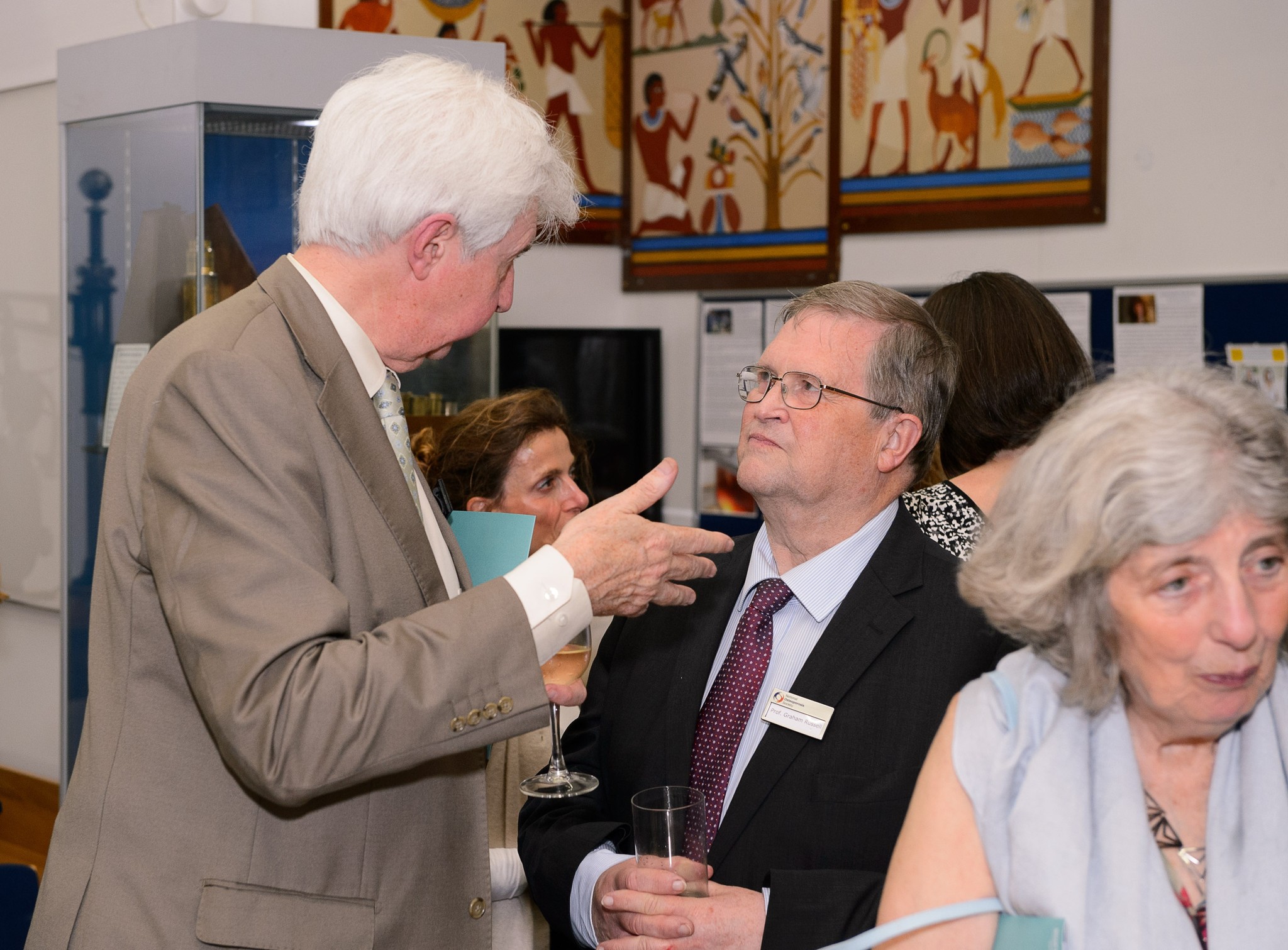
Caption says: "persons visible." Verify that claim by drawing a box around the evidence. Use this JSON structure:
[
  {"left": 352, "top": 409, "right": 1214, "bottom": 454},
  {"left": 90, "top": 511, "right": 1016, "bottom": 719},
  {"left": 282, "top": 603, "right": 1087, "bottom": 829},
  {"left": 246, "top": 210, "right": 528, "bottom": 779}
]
[
  {"left": 411, "top": 388, "right": 589, "bottom": 556},
  {"left": 869, "top": 270, "right": 1288, "bottom": 949},
  {"left": 23, "top": 51, "right": 734, "bottom": 949},
  {"left": 518, "top": 279, "right": 1024, "bottom": 949}
]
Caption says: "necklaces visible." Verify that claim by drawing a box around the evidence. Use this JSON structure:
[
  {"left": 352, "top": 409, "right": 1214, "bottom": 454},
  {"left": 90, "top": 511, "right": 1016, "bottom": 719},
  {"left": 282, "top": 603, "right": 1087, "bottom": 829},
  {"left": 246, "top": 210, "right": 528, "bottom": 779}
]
[{"left": 1146, "top": 788, "right": 1207, "bottom": 898}]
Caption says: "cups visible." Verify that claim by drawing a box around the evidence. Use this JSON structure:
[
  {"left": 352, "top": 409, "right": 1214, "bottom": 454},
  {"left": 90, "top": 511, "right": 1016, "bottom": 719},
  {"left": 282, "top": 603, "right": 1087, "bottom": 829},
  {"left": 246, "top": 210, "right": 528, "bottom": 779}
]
[{"left": 630, "top": 785, "right": 711, "bottom": 899}]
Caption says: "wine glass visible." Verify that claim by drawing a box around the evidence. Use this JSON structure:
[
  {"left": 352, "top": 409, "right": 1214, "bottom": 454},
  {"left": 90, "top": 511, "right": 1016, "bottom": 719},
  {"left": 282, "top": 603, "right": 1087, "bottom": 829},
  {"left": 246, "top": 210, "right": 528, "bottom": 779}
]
[{"left": 513, "top": 623, "right": 599, "bottom": 800}]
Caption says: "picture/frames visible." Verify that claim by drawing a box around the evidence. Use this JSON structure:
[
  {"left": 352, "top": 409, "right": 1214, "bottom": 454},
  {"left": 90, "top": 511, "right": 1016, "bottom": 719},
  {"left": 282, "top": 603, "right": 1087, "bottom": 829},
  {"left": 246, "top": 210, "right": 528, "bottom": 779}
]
[
  {"left": 615, "top": 0, "right": 840, "bottom": 293},
  {"left": 317, "top": 0, "right": 621, "bottom": 246},
  {"left": 838, "top": 0, "right": 1112, "bottom": 240}
]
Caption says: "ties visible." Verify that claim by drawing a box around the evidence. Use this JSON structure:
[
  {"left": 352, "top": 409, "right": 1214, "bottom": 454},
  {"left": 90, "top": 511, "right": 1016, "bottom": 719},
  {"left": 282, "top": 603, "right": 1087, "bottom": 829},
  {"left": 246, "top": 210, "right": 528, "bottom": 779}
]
[
  {"left": 368, "top": 372, "right": 423, "bottom": 523},
  {"left": 682, "top": 579, "right": 792, "bottom": 862}
]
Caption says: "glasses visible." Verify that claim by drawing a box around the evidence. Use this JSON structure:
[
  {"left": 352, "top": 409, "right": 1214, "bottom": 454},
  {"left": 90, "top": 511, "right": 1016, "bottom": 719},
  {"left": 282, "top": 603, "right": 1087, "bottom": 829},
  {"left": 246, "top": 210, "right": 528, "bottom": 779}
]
[{"left": 738, "top": 366, "right": 906, "bottom": 414}]
